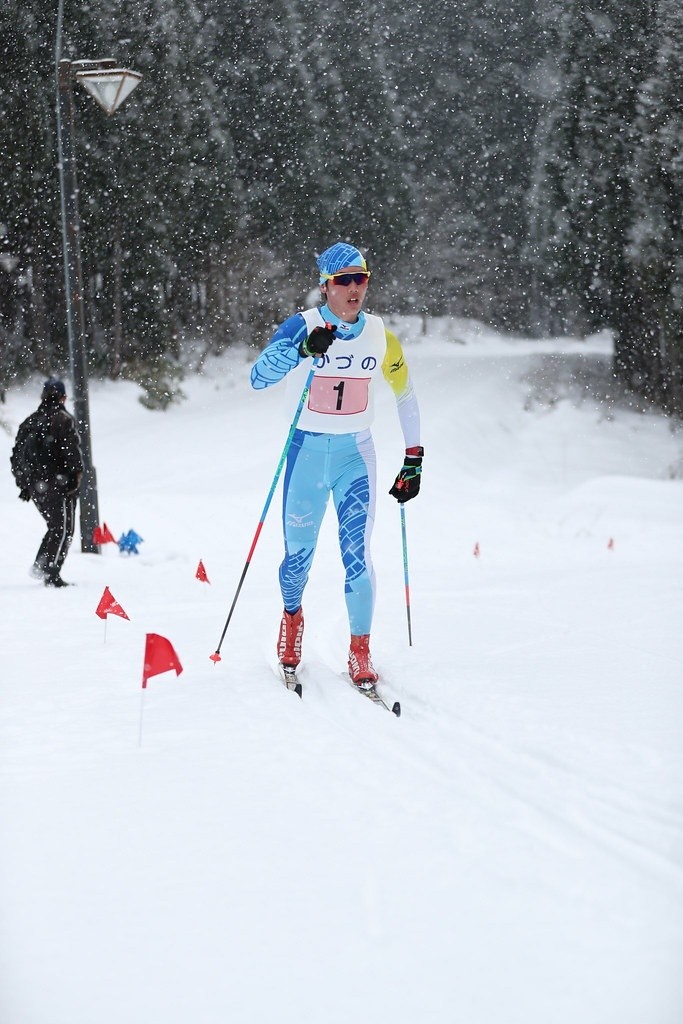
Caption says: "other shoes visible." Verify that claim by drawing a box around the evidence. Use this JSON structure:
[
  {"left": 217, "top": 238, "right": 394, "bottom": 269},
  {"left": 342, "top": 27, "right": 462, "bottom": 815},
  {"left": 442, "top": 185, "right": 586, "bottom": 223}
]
[
  {"left": 44, "top": 568, "right": 74, "bottom": 588},
  {"left": 28, "top": 560, "right": 49, "bottom": 581}
]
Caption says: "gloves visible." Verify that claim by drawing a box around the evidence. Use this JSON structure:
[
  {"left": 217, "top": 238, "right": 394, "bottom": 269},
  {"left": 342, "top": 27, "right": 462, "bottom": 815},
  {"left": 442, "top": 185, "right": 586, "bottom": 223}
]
[
  {"left": 298, "top": 325, "right": 337, "bottom": 359},
  {"left": 19, "top": 490, "right": 31, "bottom": 502},
  {"left": 65, "top": 476, "right": 80, "bottom": 495},
  {"left": 388, "top": 446, "right": 424, "bottom": 503}
]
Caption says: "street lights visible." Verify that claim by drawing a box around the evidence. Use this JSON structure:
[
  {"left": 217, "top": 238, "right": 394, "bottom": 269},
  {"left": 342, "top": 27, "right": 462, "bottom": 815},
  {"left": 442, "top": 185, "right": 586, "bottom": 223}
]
[{"left": 49, "top": 48, "right": 141, "bottom": 560}]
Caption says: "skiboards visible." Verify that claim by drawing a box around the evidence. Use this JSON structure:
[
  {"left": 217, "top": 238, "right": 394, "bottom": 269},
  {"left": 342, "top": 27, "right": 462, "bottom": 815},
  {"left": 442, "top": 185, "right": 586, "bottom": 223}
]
[{"left": 281, "top": 661, "right": 402, "bottom": 720}]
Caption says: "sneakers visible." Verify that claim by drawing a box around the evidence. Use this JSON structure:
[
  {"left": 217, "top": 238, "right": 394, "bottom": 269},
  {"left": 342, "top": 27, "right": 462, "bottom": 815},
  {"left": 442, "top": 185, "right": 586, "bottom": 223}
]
[
  {"left": 348, "top": 635, "right": 379, "bottom": 686},
  {"left": 276, "top": 605, "right": 304, "bottom": 667}
]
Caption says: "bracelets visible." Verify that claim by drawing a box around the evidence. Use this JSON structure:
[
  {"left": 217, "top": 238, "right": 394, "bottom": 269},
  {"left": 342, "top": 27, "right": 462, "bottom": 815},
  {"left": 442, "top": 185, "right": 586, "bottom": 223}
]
[
  {"left": 406, "top": 446, "right": 424, "bottom": 456},
  {"left": 302, "top": 336, "right": 317, "bottom": 357}
]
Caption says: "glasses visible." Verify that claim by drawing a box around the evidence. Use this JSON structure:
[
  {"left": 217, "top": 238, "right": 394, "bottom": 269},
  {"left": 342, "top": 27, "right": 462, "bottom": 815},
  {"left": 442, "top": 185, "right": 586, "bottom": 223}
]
[{"left": 319, "top": 271, "right": 371, "bottom": 286}]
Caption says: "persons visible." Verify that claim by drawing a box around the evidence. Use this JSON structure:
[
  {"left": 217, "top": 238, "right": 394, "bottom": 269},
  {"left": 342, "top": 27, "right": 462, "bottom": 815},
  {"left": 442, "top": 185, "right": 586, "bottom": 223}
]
[
  {"left": 10, "top": 381, "right": 85, "bottom": 587},
  {"left": 250, "top": 243, "right": 424, "bottom": 685}
]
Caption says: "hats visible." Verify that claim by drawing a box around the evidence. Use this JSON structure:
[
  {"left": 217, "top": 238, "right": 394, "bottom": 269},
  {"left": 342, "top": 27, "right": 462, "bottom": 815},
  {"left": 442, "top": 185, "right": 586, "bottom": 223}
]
[
  {"left": 40, "top": 380, "right": 65, "bottom": 402},
  {"left": 315, "top": 243, "right": 367, "bottom": 286}
]
